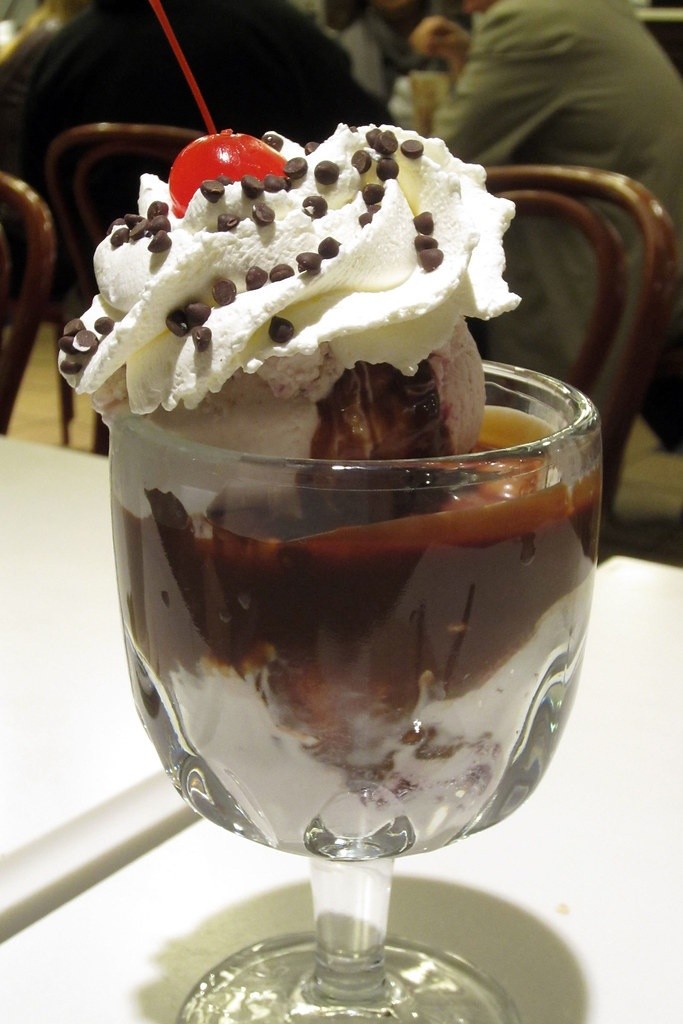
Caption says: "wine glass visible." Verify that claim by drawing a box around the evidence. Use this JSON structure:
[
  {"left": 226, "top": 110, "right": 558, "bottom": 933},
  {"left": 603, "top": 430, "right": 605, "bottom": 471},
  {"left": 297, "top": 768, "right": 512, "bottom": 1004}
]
[{"left": 109, "top": 362, "right": 601, "bottom": 1024}]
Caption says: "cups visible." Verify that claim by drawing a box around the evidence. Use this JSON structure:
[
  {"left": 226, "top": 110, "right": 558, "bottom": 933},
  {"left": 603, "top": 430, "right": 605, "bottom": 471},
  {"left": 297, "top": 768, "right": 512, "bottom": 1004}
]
[{"left": 410, "top": 69, "right": 447, "bottom": 135}]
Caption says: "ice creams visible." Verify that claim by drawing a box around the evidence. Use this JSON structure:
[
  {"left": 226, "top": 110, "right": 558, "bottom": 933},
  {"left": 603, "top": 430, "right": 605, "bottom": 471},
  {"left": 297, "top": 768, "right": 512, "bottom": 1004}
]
[{"left": 53, "top": 118, "right": 523, "bottom": 540}]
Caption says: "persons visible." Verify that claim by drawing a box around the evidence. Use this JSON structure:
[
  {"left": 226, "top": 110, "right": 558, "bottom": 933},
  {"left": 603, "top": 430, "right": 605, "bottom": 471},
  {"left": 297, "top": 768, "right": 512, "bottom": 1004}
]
[{"left": 0, "top": 0, "right": 683, "bottom": 442}]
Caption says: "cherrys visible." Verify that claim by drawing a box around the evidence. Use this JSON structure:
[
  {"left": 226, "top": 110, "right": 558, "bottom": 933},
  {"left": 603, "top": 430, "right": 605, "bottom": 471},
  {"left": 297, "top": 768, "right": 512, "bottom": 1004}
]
[{"left": 148, "top": 0, "right": 294, "bottom": 211}]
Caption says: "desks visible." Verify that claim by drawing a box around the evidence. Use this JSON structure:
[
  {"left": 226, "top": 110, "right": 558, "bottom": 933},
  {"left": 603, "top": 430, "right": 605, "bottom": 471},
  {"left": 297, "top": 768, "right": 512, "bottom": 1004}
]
[{"left": 0, "top": 430, "right": 683, "bottom": 1024}]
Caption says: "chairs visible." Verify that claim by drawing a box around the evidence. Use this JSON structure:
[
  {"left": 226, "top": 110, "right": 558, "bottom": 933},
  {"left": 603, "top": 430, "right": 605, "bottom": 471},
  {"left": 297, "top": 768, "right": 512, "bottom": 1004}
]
[
  {"left": 44, "top": 123, "right": 204, "bottom": 456},
  {"left": 0, "top": 173, "right": 56, "bottom": 435},
  {"left": 463, "top": 168, "right": 670, "bottom": 565}
]
